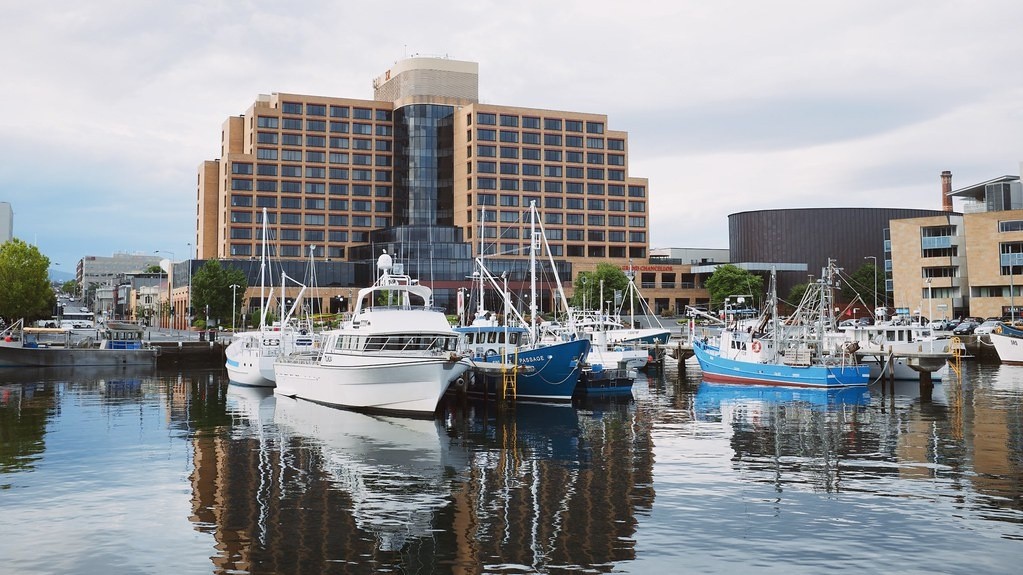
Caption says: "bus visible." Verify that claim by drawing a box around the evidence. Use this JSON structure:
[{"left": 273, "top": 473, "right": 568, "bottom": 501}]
[{"left": 39, "top": 313, "right": 96, "bottom": 329}]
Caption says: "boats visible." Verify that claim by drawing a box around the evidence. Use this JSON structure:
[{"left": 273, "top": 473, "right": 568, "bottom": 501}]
[
  {"left": 692, "top": 375, "right": 873, "bottom": 507},
  {"left": 0, "top": 317, "right": 158, "bottom": 366},
  {"left": 267, "top": 391, "right": 450, "bottom": 549},
  {"left": 273, "top": 249, "right": 480, "bottom": 418},
  {"left": 684, "top": 260, "right": 969, "bottom": 392}
]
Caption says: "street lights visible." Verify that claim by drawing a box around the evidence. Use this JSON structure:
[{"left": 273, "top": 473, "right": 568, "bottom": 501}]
[
  {"left": 228, "top": 283, "right": 241, "bottom": 333},
  {"left": 864, "top": 256, "right": 877, "bottom": 308},
  {"left": 187, "top": 242, "right": 191, "bottom": 341},
  {"left": 155, "top": 251, "right": 175, "bottom": 334}
]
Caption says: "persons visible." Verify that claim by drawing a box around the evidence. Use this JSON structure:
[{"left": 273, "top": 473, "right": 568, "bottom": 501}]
[{"left": 327, "top": 318, "right": 333, "bottom": 331}]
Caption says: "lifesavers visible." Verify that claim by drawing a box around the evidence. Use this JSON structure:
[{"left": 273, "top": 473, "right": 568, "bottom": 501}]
[{"left": 752, "top": 341, "right": 761, "bottom": 353}]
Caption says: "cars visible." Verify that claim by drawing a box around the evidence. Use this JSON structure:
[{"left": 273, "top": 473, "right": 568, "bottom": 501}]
[
  {"left": 686, "top": 307, "right": 715, "bottom": 319},
  {"left": 953, "top": 322, "right": 979, "bottom": 336},
  {"left": 974, "top": 321, "right": 1001, "bottom": 334},
  {"left": 840, "top": 316, "right": 1002, "bottom": 332},
  {"left": 54, "top": 289, "right": 89, "bottom": 313}
]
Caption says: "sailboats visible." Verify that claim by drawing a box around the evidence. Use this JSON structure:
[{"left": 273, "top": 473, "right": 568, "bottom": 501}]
[
  {"left": 224, "top": 380, "right": 288, "bottom": 546},
  {"left": 989, "top": 245, "right": 1023, "bottom": 365},
  {"left": 225, "top": 207, "right": 329, "bottom": 387},
  {"left": 451, "top": 197, "right": 672, "bottom": 410}
]
